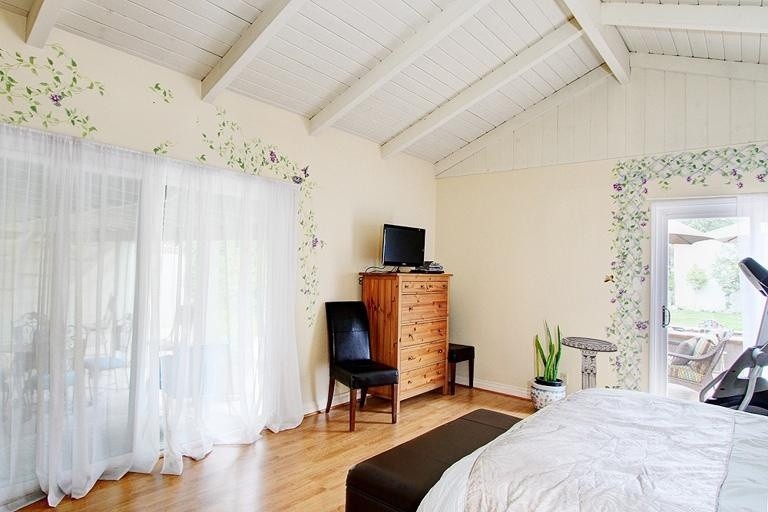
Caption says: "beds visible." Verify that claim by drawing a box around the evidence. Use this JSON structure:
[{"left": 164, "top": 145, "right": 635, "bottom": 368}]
[{"left": 415, "top": 387, "right": 768, "bottom": 511}]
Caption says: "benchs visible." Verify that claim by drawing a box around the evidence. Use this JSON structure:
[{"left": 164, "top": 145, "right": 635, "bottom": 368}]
[{"left": 346, "top": 409, "right": 524, "bottom": 511}]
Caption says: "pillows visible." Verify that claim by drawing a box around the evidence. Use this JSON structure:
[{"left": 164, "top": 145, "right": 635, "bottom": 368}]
[{"left": 671, "top": 335, "right": 701, "bottom": 364}]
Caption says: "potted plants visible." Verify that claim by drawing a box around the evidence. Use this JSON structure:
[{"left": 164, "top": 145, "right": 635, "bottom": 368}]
[{"left": 531, "top": 320, "right": 567, "bottom": 411}]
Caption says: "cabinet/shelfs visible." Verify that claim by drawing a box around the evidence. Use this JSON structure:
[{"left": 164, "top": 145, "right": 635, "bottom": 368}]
[{"left": 361, "top": 273, "right": 453, "bottom": 412}]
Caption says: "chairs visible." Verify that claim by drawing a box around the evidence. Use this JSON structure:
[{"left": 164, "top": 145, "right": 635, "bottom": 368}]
[
  {"left": 325, "top": 301, "right": 398, "bottom": 432},
  {"left": 667, "top": 319, "right": 733, "bottom": 393}
]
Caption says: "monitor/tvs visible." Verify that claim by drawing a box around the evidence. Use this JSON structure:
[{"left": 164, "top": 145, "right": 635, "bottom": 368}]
[{"left": 382, "top": 224, "right": 425, "bottom": 267}]
